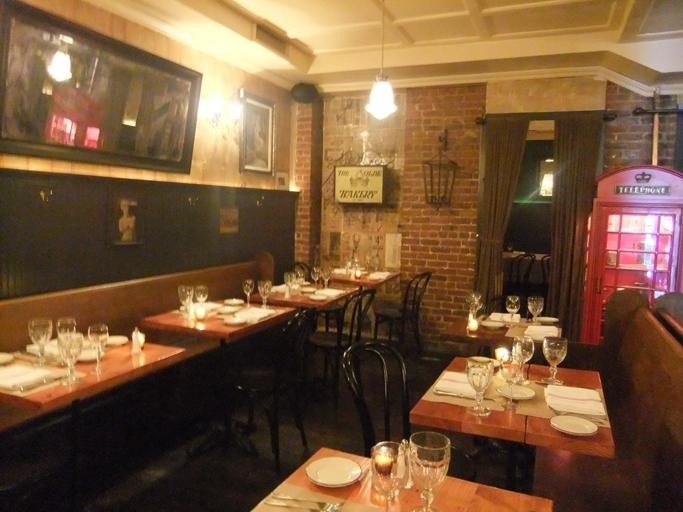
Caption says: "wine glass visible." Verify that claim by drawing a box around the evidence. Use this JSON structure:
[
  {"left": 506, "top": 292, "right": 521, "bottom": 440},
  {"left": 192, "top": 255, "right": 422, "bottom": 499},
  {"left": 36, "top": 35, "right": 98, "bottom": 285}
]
[
  {"left": 466, "top": 358, "right": 490, "bottom": 417},
  {"left": 27, "top": 319, "right": 52, "bottom": 370},
  {"left": 273, "top": 261, "right": 340, "bottom": 302},
  {"left": 527, "top": 296, "right": 543, "bottom": 325},
  {"left": 513, "top": 336, "right": 534, "bottom": 384},
  {"left": 541, "top": 336, "right": 567, "bottom": 384},
  {"left": 87, "top": 325, "right": 109, "bottom": 374},
  {"left": 242, "top": 280, "right": 254, "bottom": 309},
  {"left": 407, "top": 431, "right": 450, "bottom": 512},
  {"left": 59, "top": 334, "right": 82, "bottom": 384},
  {"left": 257, "top": 281, "right": 272, "bottom": 310},
  {"left": 57, "top": 319, "right": 76, "bottom": 365},
  {"left": 500, "top": 359, "right": 518, "bottom": 411},
  {"left": 506, "top": 296, "right": 519, "bottom": 327},
  {"left": 369, "top": 440, "right": 407, "bottom": 512}
]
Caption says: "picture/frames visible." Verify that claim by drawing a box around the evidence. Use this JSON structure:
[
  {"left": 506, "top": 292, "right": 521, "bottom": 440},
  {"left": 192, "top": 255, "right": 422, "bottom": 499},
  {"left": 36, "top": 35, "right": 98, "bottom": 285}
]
[{"left": 239, "top": 88, "right": 277, "bottom": 177}]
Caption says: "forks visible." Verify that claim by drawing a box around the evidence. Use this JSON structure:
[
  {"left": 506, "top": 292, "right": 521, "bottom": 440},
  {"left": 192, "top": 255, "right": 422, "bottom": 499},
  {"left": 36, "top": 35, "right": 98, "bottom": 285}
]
[{"left": 263, "top": 495, "right": 340, "bottom": 512}]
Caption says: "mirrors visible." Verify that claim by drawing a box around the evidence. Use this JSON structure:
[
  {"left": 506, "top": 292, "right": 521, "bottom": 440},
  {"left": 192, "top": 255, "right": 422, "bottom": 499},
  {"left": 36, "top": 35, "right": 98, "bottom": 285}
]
[{"left": 0, "top": 0, "right": 203, "bottom": 175}]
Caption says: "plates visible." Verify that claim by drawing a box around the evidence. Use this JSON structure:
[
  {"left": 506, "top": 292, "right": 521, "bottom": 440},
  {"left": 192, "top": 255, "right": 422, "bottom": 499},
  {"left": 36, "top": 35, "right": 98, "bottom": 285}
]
[
  {"left": 479, "top": 311, "right": 562, "bottom": 340},
  {"left": 174, "top": 283, "right": 246, "bottom": 328},
  {"left": 549, "top": 414, "right": 601, "bottom": 438},
  {"left": 305, "top": 457, "right": 361, "bottom": 487},
  {"left": 0, "top": 334, "right": 128, "bottom": 393},
  {"left": 494, "top": 382, "right": 538, "bottom": 402}
]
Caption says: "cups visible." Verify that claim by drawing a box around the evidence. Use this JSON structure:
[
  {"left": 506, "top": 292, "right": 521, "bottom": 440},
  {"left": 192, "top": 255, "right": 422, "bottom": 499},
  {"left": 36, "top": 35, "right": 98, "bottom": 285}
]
[{"left": 340, "top": 254, "right": 381, "bottom": 281}]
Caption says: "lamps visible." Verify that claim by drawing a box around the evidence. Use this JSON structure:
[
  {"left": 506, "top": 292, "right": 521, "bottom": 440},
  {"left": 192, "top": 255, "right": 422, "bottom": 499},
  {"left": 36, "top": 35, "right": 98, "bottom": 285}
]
[
  {"left": 365, "top": 0, "right": 398, "bottom": 121},
  {"left": 47, "top": 42, "right": 72, "bottom": 82},
  {"left": 538, "top": 159, "right": 555, "bottom": 198}
]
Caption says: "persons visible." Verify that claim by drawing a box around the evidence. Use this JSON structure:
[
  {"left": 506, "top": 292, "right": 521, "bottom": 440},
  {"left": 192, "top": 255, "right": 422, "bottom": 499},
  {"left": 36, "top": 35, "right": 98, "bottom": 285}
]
[{"left": 117, "top": 198, "right": 137, "bottom": 242}]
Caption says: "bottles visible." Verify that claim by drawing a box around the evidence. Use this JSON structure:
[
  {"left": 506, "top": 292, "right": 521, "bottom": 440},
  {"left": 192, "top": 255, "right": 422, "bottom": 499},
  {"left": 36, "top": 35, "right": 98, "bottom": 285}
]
[{"left": 131, "top": 325, "right": 146, "bottom": 356}]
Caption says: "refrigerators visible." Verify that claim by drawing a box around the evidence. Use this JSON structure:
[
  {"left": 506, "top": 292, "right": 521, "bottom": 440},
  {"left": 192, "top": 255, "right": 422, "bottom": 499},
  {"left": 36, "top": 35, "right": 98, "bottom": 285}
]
[{"left": 580, "top": 160, "right": 679, "bottom": 350}]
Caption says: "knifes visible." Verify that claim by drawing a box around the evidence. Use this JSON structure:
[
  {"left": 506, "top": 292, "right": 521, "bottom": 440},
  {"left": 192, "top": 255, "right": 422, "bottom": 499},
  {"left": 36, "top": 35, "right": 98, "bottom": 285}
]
[{"left": 433, "top": 389, "right": 493, "bottom": 401}]
